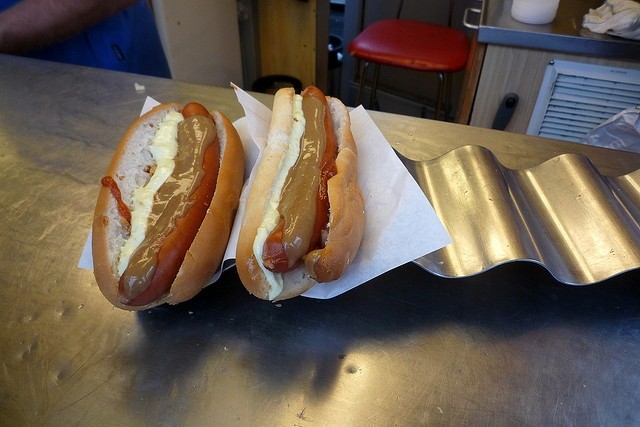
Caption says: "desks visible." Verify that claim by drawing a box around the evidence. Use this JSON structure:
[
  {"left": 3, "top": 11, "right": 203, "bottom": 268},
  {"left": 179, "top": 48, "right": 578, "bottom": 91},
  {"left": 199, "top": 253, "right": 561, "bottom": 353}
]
[{"left": 0, "top": 53, "right": 639, "bottom": 426}]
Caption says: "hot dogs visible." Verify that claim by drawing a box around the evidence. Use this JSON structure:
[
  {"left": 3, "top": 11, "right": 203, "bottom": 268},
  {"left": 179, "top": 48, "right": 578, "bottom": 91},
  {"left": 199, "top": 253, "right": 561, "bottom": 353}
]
[
  {"left": 235, "top": 87, "right": 365, "bottom": 302},
  {"left": 90, "top": 102, "right": 245, "bottom": 311}
]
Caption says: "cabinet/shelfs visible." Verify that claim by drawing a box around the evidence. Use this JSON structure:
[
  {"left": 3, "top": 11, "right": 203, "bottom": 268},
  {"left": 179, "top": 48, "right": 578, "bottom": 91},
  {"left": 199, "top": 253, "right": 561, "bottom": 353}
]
[{"left": 457, "top": 0, "right": 639, "bottom": 153}]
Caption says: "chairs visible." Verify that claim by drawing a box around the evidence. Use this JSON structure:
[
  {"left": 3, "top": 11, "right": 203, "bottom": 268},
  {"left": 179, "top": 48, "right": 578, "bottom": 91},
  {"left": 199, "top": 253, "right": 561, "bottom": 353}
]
[{"left": 349, "top": 1, "right": 469, "bottom": 120}]
